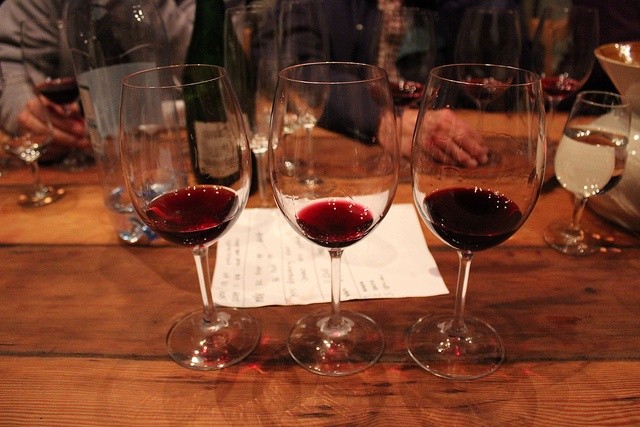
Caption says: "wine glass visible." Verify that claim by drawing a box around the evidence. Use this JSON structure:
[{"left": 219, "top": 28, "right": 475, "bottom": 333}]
[
  {"left": 118, "top": 63, "right": 261, "bottom": 371},
  {"left": 543, "top": 90, "right": 632, "bottom": 257},
  {"left": 223, "top": 4, "right": 283, "bottom": 208},
  {"left": 272, "top": 0, "right": 338, "bottom": 198},
  {"left": 20, "top": 27, "right": 93, "bottom": 172},
  {"left": 406, "top": 65, "right": 548, "bottom": 380},
  {"left": 267, "top": 60, "right": 400, "bottom": 378},
  {"left": 2, "top": 65, "right": 68, "bottom": 207}
]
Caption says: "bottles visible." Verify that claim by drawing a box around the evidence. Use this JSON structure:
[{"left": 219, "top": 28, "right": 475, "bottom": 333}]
[{"left": 61, "top": 2, "right": 195, "bottom": 242}]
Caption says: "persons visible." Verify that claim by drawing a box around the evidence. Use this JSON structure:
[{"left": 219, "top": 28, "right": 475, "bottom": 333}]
[{"left": 1, "top": 1, "right": 530, "bottom": 169}]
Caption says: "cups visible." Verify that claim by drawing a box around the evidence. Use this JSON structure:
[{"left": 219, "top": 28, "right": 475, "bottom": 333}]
[{"left": 278, "top": 66, "right": 330, "bottom": 183}]
